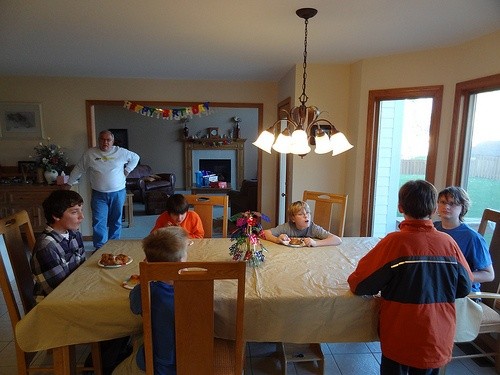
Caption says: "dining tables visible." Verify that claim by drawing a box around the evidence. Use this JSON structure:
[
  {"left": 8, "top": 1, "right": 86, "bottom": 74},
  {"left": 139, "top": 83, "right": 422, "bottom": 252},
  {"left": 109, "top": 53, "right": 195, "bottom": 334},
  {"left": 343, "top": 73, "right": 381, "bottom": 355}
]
[{"left": 16, "top": 232, "right": 482, "bottom": 375}]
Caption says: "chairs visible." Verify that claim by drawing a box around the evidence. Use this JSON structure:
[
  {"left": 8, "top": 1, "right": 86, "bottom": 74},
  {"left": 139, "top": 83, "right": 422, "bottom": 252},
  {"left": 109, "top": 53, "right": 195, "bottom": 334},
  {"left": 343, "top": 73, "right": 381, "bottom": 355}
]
[
  {"left": 138, "top": 261, "right": 246, "bottom": 375},
  {"left": 0, "top": 209, "right": 100, "bottom": 375},
  {"left": 184, "top": 195, "right": 228, "bottom": 238},
  {"left": 433, "top": 208, "right": 500, "bottom": 375},
  {"left": 303, "top": 191, "right": 348, "bottom": 237}
]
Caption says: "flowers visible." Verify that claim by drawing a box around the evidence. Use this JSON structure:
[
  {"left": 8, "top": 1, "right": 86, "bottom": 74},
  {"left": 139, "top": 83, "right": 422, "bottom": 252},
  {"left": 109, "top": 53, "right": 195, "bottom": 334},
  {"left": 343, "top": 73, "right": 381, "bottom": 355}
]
[{"left": 33, "top": 142, "right": 65, "bottom": 172}]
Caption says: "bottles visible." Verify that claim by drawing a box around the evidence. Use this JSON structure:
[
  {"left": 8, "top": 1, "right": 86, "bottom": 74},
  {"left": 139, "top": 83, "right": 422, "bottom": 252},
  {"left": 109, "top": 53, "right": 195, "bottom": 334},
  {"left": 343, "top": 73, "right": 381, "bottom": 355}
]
[
  {"left": 61, "top": 171, "right": 65, "bottom": 176},
  {"left": 195, "top": 171, "right": 202, "bottom": 187}
]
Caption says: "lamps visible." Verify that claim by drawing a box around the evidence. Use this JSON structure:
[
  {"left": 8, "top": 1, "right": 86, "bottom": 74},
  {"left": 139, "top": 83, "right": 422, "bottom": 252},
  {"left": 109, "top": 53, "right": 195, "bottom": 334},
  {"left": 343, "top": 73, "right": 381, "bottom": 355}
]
[{"left": 252, "top": 7, "right": 354, "bottom": 158}]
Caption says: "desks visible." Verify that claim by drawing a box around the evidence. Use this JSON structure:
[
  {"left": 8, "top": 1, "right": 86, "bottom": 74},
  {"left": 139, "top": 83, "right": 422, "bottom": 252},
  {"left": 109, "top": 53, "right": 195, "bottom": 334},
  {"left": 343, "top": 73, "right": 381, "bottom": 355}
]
[{"left": 123, "top": 191, "right": 134, "bottom": 228}]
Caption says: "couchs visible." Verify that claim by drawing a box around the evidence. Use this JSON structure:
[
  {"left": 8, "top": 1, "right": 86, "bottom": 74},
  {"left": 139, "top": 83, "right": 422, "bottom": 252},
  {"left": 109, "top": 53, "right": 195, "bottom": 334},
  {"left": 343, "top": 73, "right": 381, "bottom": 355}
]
[
  {"left": 227, "top": 178, "right": 257, "bottom": 212},
  {"left": 126, "top": 173, "right": 176, "bottom": 215}
]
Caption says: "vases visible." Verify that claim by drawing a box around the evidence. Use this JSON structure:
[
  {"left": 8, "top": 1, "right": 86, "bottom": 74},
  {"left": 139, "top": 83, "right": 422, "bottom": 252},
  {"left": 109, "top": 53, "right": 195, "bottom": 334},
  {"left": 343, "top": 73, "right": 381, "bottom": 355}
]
[{"left": 44, "top": 167, "right": 58, "bottom": 184}]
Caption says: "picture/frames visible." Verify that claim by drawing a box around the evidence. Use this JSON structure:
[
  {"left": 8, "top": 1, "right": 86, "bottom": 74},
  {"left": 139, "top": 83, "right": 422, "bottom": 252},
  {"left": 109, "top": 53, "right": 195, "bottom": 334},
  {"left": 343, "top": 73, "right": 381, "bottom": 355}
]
[{"left": 0, "top": 102, "right": 45, "bottom": 140}]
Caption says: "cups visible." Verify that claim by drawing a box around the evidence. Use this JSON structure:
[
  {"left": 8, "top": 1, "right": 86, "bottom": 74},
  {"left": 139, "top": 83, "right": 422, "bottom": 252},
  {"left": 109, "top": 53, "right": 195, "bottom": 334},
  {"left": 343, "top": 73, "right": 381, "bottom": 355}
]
[{"left": 34, "top": 167, "right": 43, "bottom": 184}]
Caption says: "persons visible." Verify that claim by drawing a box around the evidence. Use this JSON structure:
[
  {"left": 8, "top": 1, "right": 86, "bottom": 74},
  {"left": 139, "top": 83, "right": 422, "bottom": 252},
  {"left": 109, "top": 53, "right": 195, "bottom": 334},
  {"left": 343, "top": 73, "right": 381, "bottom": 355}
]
[
  {"left": 129, "top": 226, "right": 190, "bottom": 375},
  {"left": 61, "top": 130, "right": 139, "bottom": 255},
  {"left": 150, "top": 194, "right": 204, "bottom": 239},
  {"left": 347, "top": 180, "right": 473, "bottom": 375},
  {"left": 259, "top": 201, "right": 342, "bottom": 247},
  {"left": 32, "top": 190, "right": 130, "bottom": 375},
  {"left": 432, "top": 186, "right": 495, "bottom": 303}
]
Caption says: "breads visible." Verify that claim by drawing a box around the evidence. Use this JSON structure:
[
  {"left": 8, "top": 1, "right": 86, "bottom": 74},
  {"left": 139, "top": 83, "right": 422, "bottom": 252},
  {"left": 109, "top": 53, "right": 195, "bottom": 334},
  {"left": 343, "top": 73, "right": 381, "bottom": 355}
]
[
  {"left": 289, "top": 239, "right": 303, "bottom": 245},
  {"left": 100, "top": 253, "right": 128, "bottom": 266}
]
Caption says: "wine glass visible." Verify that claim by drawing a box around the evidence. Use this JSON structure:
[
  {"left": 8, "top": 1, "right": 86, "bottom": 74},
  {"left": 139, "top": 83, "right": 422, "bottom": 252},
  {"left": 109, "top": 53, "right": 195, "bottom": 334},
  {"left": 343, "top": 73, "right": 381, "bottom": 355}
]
[{"left": 21, "top": 163, "right": 30, "bottom": 184}]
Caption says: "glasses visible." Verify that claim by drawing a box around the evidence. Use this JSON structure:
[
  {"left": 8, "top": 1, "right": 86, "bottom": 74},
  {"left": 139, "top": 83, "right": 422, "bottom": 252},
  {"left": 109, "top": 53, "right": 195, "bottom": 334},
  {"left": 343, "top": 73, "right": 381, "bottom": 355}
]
[
  {"left": 296, "top": 211, "right": 311, "bottom": 216},
  {"left": 437, "top": 200, "right": 460, "bottom": 207}
]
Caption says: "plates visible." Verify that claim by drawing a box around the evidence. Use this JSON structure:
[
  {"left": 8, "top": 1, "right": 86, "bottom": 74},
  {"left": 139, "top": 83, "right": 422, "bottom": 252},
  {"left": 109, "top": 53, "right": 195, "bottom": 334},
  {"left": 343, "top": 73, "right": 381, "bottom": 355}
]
[
  {"left": 288, "top": 238, "right": 304, "bottom": 247},
  {"left": 96, "top": 255, "right": 133, "bottom": 269},
  {"left": 124, "top": 280, "right": 134, "bottom": 289}
]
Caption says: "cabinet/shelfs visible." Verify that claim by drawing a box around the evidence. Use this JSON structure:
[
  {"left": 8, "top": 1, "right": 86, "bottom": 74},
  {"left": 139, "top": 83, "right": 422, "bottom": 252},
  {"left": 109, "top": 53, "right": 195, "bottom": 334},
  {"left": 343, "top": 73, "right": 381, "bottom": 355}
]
[{"left": 0, "top": 180, "right": 79, "bottom": 242}]
